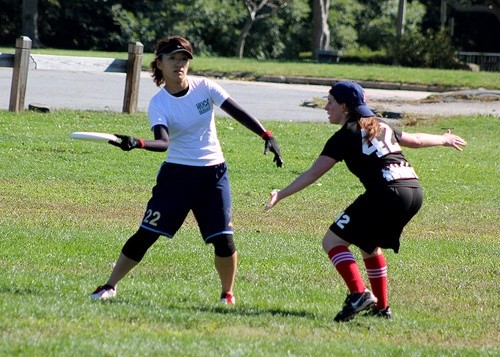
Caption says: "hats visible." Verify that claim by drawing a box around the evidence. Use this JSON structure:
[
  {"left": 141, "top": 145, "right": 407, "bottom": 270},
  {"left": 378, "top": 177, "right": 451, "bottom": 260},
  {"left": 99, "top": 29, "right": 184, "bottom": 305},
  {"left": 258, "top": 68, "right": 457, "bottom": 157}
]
[
  {"left": 156, "top": 44, "right": 193, "bottom": 60},
  {"left": 329, "top": 81, "right": 374, "bottom": 118}
]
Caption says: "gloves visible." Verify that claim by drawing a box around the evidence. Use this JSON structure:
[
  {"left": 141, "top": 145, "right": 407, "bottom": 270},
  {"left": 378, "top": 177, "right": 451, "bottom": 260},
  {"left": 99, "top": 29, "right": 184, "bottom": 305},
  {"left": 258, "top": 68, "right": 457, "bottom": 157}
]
[
  {"left": 109, "top": 133, "right": 144, "bottom": 151},
  {"left": 261, "top": 131, "right": 286, "bottom": 169}
]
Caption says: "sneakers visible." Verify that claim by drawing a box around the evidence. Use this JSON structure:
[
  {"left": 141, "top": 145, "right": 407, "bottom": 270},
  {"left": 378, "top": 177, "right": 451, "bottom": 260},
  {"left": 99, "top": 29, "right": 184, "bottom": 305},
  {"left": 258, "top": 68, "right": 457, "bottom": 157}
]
[
  {"left": 333, "top": 288, "right": 378, "bottom": 322},
  {"left": 361, "top": 304, "right": 392, "bottom": 319},
  {"left": 88, "top": 284, "right": 116, "bottom": 299},
  {"left": 220, "top": 294, "right": 235, "bottom": 305}
]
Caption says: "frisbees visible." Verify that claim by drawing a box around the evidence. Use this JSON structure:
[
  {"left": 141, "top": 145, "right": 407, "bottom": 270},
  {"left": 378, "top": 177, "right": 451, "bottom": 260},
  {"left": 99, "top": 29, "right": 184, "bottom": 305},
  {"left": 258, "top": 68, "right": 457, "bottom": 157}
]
[{"left": 68, "top": 131, "right": 118, "bottom": 142}]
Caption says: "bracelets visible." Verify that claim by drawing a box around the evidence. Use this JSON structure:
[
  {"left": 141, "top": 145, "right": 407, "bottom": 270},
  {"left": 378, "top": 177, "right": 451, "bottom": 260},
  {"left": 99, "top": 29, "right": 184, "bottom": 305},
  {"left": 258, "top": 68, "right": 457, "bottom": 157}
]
[
  {"left": 262, "top": 131, "right": 272, "bottom": 141},
  {"left": 135, "top": 138, "right": 145, "bottom": 149}
]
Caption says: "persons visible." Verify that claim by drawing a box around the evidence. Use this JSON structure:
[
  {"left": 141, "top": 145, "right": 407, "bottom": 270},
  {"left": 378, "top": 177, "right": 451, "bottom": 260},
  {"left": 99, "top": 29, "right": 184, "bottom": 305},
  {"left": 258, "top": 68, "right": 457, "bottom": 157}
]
[
  {"left": 261, "top": 80, "right": 468, "bottom": 323},
  {"left": 89, "top": 35, "right": 283, "bottom": 304}
]
